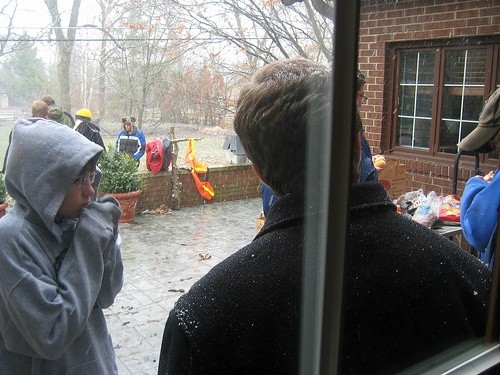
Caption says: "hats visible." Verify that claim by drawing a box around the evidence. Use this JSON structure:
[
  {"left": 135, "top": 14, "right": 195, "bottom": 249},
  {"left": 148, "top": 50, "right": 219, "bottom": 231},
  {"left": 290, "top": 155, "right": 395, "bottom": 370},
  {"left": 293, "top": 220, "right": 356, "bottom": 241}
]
[
  {"left": 457, "top": 87, "right": 500, "bottom": 151},
  {"left": 122, "top": 117, "right": 136, "bottom": 124},
  {"left": 48, "top": 107, "right": 72, "bottom": 127}
]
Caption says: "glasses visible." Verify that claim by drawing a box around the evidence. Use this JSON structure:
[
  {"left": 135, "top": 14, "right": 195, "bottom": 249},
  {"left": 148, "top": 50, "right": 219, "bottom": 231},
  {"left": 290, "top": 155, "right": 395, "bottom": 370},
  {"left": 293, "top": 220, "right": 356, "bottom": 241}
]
[
  {"left": 356, "top": 93, "right": 367, "bottom": 104},
  {"left": 76, "top": 172, "right": 95, "bottom": 188}
]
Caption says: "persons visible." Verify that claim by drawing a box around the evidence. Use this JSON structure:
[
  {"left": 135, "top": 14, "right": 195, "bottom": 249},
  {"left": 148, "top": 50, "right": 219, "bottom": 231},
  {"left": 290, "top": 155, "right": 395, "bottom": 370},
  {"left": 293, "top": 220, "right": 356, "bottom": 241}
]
[
  {"left": 458, "top": 89, "right": 500, "bottom": 271},
  {"left": 157, "top": 59, "right": 500, "bottom": 375},
  {"left": 0, "top": 118, "right": 123, "bottom": 375},
  {"left": 3, "top": 96, "right": 146, "bottom": 175},
  {"left": 261, "top": 64, "right": 377, "bottom": 217}
]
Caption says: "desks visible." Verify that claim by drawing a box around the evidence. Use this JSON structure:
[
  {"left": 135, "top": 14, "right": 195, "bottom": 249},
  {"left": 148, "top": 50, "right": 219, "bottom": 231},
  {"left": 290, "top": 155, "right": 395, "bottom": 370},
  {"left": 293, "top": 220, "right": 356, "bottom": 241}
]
[{"left": 434, "top": 225, "right": 474, "bottom": 255}]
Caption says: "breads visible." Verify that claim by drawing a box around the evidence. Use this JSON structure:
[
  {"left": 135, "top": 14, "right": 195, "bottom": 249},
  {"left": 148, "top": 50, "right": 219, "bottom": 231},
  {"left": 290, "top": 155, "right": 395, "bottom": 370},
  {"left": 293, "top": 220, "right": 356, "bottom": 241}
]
[{"left": 372, "top": 154, "right": 386, "bottom": 168}]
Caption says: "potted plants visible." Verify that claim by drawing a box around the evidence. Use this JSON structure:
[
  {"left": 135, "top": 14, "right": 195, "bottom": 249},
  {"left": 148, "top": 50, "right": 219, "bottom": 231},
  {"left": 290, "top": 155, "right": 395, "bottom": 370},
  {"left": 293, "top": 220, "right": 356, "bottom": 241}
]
[
  {"left": 0, "top": 173, "right": 8, "bottom": 219},
  {"left": 99, "top": 140, "right": 145, "bottom": 223}
]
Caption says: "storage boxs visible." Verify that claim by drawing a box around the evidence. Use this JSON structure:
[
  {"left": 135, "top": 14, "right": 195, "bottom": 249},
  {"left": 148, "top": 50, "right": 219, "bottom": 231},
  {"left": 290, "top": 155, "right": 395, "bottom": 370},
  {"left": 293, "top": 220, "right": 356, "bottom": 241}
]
[
  {"left": 255, "top": 216, "right": 266, "bottom": 234},
  {"left": 378, "top": 159, "right": 406, "bottom": 203}
]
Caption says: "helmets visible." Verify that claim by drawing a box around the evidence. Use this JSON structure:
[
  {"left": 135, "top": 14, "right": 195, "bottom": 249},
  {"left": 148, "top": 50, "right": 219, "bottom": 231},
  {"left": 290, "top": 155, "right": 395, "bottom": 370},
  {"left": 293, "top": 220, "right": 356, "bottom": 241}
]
[{"left": 75, "top": 109, "right": 92, "bottom": 121}]
